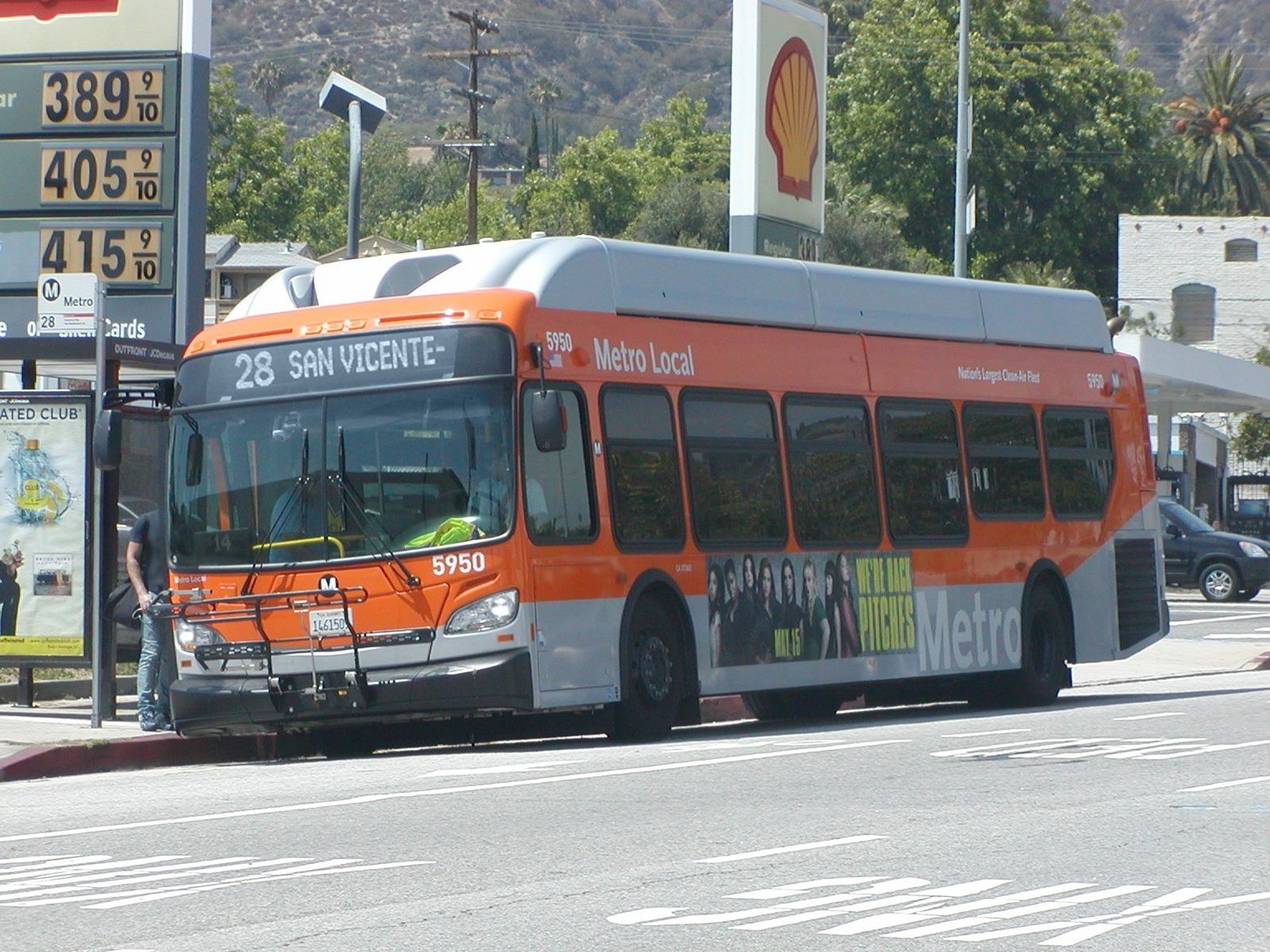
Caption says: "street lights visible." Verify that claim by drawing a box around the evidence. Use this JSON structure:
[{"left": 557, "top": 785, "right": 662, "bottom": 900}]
[{"left": 314, "top": 72, "right": 393, "bottom": 258}]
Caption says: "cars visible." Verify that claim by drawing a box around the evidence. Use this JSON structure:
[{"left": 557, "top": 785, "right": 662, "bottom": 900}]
[{"left": 1156, "top": 496, "right": 1270, "bottom": 606}]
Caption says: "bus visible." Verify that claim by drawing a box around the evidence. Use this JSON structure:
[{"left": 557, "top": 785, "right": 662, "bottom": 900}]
[{"left": 89, "top": 227, "right": 1175, "bottom": 761}]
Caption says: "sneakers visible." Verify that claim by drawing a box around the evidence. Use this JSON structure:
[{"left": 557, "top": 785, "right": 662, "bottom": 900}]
[
  {"left": 155, "top": 711, "right": 173, "bottom": 730},
  {"left": 139, "top": 712, "right": 158, "bottom": 730}
]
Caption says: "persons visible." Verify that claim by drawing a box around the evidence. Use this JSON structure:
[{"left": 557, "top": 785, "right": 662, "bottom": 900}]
[
  {"left": 127, "top": 510, "right": 178, "bottom": 730},
  {"left": 0, "top": 546, "right": 25, "bottom": 635},
  {"left": 708, "top": 552, "right": 861, "bottom": 664},
  {"left": 269, "top": 448, "right": 549, "bottom": 568}
]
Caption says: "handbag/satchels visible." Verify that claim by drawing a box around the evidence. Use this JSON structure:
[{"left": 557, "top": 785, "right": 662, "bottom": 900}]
[{"left": 103, "top": 580, "right": 143, "bottom": 630}]
